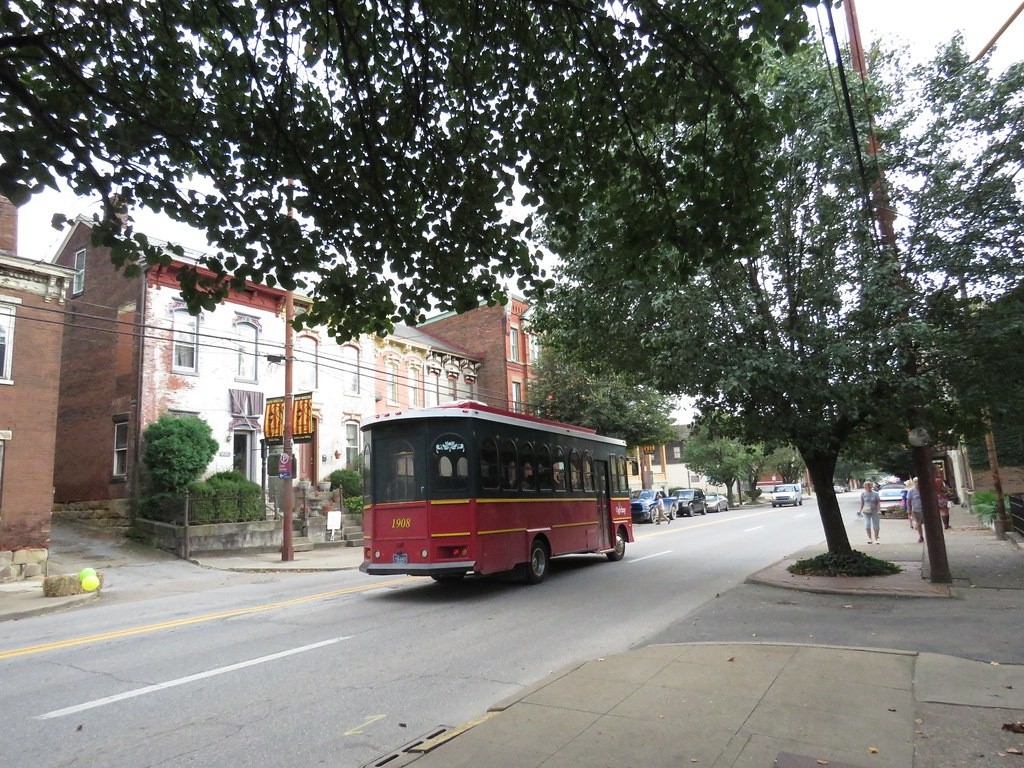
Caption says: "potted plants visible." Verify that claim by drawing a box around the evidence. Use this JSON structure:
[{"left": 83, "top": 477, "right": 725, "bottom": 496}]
[
  {"left": 318, "top": 476, "right": 331, "bottom": 492},
  {"left": 332, "top": 489, "right": 340, "bottom": 503},
  {"left": 297, "top": 473, "right": 312, "bottom": 490}
]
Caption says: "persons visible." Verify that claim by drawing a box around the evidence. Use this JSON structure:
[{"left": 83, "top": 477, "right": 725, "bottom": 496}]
[
  {"left": 653, "top": 494, "right": 670, "bottom": 525},
  {"left": 901, "top": 477, "right": 925, "bottom": 543},
  {"left": 935, "top": 478, "right": 951, "bottom": 529},
  {"left": 859, "top": 481, "right": 881, "bottom": 544},
  {"left": 873, "top": 482, "right": 881, "bottom": 492}
]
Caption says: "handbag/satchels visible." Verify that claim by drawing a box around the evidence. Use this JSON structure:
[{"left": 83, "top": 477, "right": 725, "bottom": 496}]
[
  {"left": 947, "top": 500, "right": 955, "bottom": 508},
  {"left": 861, "top": 505, "right": 872, "bottom": 514}
]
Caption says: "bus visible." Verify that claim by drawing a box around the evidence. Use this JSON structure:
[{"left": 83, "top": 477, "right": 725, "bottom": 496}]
[{"left": 358, "top": 401, "right": 634, "bottom": 584}]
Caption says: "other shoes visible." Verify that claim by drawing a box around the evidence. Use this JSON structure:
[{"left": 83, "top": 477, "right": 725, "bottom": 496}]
[
  {"left": 945, "top": 525, "right": 951, "bottom": 529},
  {"left": 655, "top": 523, "right": 660, "bottom": 525},
  {"left": 668, "top": 519, "right": 670, "bottom": 524},
  {"left": 918, "top": 536, "right": 924, "bottom": 543},
  {"left": 875, "top": 537, "right": 880, "bottom": 544},
  {"left": 867, "top": 538, "right": 872, "bottom": 544},
  {"left": 910, "top": 525, "right": 918, "bottom": 530}
]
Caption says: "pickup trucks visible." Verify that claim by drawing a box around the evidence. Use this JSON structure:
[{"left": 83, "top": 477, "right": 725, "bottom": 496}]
[{"left": 630, "top": 490, "right": 678, "bottom": 524}]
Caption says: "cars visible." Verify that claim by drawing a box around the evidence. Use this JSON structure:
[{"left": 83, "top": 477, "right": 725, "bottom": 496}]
[
  {"left": 705, "top": 493, "right": 730, "bottom": 512},
  {"left": 877, "top": 485, "right": 906, "bottom": 514}
]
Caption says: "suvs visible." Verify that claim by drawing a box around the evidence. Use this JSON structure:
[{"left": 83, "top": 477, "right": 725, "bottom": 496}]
[{"left": 671, "top": 489, "right": 706, "bottom": 516}]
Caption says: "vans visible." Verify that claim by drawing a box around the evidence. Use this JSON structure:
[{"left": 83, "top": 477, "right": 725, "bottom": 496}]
[{"left": 771, "top": 485, "right": 803, "bottom": 508}]
